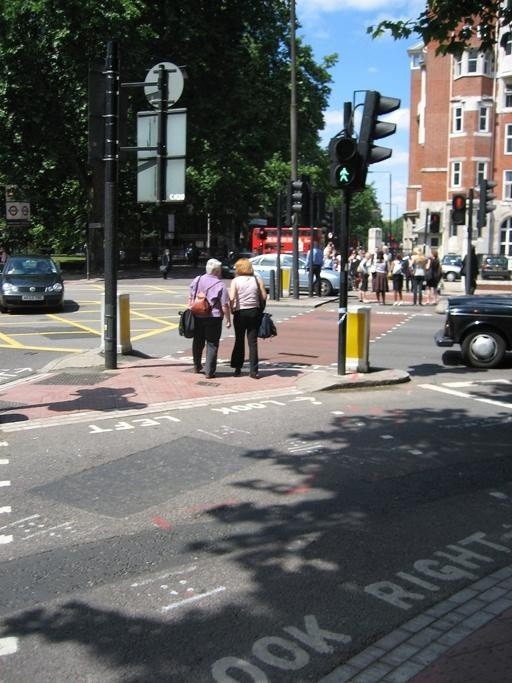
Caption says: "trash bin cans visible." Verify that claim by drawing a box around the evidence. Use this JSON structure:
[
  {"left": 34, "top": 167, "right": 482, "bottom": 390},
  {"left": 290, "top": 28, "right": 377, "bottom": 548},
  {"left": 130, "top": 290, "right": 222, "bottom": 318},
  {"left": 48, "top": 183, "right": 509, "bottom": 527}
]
[
  {"left": 345, "top": 305, "right": 371, "bottom": 373},
  {"left": 101, "top": 292, "right": 132, "bottom": 355},
  {"left": 282, "top": 268, "right": 291, "bottom": 297}
]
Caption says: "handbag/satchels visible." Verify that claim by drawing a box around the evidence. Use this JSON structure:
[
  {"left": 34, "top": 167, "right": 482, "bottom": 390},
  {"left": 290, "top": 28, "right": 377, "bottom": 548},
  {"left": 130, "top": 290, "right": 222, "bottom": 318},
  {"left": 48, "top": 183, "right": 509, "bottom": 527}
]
[
  {"left": 257, "top": 296, "right": 266, "bottom": 312},
  {"left": 368, "top": 265, "right": 376, "bottom": 274},
  {"left": 356, "top": 264, "right": 364, "bottom": 273},
  {"left": 178, "top": 308, "right": 198, "bottom": 339},
  {"left": 160, "top": 265, "right": 168, "bottom": 274},
  {"left": 393, "top": 264, "right": 403, "bottom": 274}
]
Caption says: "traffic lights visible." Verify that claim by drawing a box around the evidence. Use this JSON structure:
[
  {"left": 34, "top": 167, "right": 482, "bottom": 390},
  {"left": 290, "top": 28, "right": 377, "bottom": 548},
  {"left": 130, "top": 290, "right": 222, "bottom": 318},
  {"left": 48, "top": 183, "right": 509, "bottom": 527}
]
[
  {"left": 321, "top": 210, "right": 334, "bottom": 236},
  {"left": 256, "top": 227, "right": 267, "bottom": 239},
  {"left": 314, "top": 194, "right": 329, "bottom": 225},
  {"left": 329, "top": 138, "right": 360, "bottom": 191},
  {"left": 359, "top": 91, "right": 400, "bottom": 165},
  {"left": 290, "top": 179, "right": 310, "bottom": 214},
  {"left": 480, "top": 178, "right": 498, "bottom": 215},
  {"left": 430, "top": 211, "right": 441, "bottom": 232},
  {"left": 451, "top": 193, "right": 468, "bottom": 225}
]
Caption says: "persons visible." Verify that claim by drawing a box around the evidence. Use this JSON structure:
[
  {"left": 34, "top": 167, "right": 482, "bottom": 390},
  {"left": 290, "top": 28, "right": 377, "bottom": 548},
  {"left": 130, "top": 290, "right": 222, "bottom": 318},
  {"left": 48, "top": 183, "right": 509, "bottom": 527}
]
[
  {"left": 304, "top": 239, "right": 442, "bottom": 306},
  {"left": 8, "top": 262, "right": 22, "bottom": 274},
  {"left": 187, "top": 259, "right": 231, "bottom": 379},
  {"left": 38, "top": 262, "right": 48, "bottom": 271},
  {"left": 161, "top": 248, "right": 171, "bottom": 280},
  {"left": 227, "top": 258, "right": 267, "bottom": 379},
  {"left": 1, "top": 246, "right": 10, "bottom": 264}
]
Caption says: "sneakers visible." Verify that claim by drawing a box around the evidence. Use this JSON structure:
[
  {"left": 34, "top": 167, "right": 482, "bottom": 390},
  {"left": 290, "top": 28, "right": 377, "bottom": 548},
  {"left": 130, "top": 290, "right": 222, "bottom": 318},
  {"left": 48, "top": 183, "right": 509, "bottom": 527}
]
[
  {"left": 232, "top": 369, "right": 257, "bottom": 379},
  {"left": 359, "top": 299, "right": 386, "bottom": 306},
  {"left": 411, "top": 301, "right": 438, "bottom": 307},
  {"left": 193, "top": 365, "right": 203, "bottom": 373},
  {"left": 393, "top": 301, "right": 404, "bottom": 307},
  {"left": 316, "top": 291, "right": 321, "bottom": 297},
  {"left": 205, "top": 372, "right": 216, "bottom": 379}
]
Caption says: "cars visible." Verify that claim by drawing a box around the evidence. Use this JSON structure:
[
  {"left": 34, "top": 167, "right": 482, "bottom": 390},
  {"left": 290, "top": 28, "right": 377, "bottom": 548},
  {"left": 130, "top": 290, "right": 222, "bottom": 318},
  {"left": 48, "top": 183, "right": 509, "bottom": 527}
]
[
  {"left": 229, "top": 253, "right": 353, "bottom": 297},
  {"left": 390, "top": 255, "right": 462, "bottom": 282},
  {"left": 222, "top": 251, "right": 255, "bottom": 277},
  {"left": 0, "top": 256, "right": 65, "bottom": 314},
  {"left": 440, "top": 253, "right": 464, "bottom": 266},
  {"left": 434, "top": 292, "right": 512, "bottom": 366}
]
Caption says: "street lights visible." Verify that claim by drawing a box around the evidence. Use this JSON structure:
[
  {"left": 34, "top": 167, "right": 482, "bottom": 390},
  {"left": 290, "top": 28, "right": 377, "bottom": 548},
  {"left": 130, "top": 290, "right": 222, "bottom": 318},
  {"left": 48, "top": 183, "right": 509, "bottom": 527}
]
[
  {"left": 366, "top": 170, "right": 393, "bottom": 250},
  {"left": 384, "top": 202, "right": 400, "bottom": 242}
]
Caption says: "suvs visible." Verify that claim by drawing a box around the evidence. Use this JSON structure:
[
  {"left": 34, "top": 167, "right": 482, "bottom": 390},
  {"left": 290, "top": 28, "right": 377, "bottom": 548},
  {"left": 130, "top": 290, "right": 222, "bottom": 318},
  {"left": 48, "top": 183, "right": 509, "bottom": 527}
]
[{"left": 481, "top": 254, "right": 508, "bottom": 280}]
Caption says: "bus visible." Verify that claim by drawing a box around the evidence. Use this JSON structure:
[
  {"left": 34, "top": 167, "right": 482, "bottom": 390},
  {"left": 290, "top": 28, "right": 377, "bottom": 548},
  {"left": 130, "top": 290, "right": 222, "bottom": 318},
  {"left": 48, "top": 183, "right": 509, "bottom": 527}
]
[{"left": 252, "top": 226, "right": 328, "bottom": 255}]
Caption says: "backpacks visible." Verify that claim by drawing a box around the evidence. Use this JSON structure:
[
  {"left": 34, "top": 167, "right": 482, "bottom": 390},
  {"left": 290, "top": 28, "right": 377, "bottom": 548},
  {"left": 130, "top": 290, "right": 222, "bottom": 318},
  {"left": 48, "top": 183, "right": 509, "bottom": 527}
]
[
  {"left": 257, "top": 312, "right": 276, "bottom": 339},
  {"left": 191, "top": 291, "right": 213, "bottom": 316}
]
